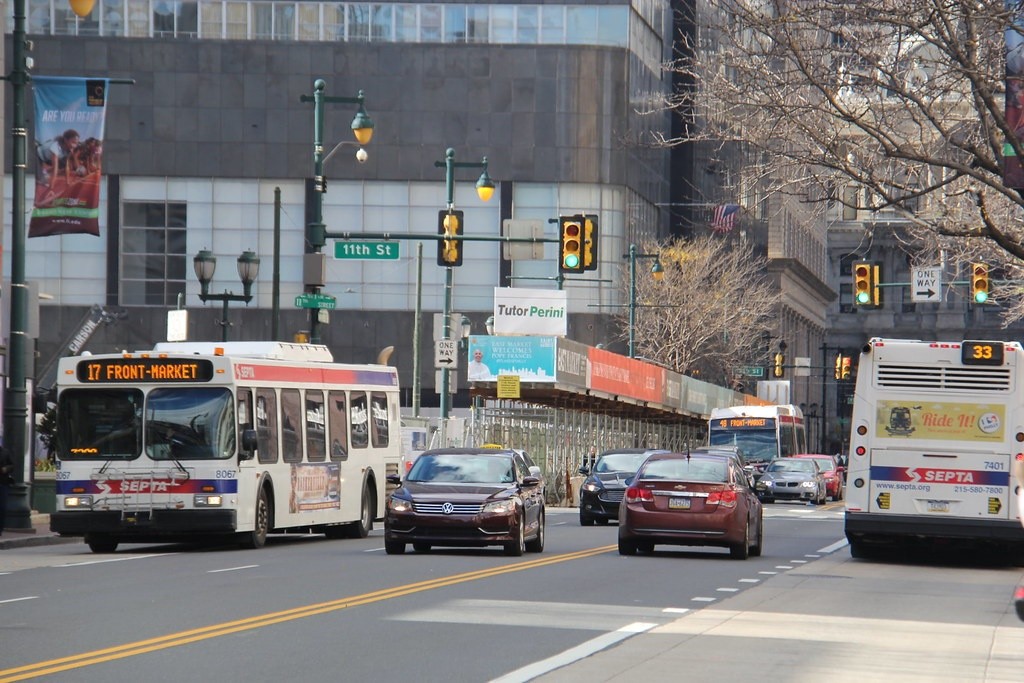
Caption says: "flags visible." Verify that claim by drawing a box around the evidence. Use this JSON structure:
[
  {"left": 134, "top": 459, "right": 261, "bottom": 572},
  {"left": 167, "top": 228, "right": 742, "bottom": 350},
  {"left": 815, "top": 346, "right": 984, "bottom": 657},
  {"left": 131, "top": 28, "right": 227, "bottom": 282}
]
[{"left": 708, "top": 204, "right": 740, "bottom": 233}]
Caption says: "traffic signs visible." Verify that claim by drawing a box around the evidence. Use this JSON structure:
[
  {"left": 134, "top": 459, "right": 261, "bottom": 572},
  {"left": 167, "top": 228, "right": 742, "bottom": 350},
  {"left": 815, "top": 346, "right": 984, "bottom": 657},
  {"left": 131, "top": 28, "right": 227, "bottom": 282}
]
[
  {"left": 333, "top": 241, "right": 401, "bottom": 261},
  {"left": 911, "top": 267, "right": 942, "bottom": 303},
  {"left": 435, "top": 340, "right": 459, "bottom": 371},
  {"left": 731, "top": 366, "right": 763, "bottom": 376}
]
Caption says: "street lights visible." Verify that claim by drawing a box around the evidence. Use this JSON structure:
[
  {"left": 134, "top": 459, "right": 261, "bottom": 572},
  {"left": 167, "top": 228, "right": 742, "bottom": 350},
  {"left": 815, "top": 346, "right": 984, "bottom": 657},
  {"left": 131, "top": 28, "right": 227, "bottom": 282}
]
[
  {"left": 193, "top": 246, "right": 260, "bottom": 342},
  {"left": 299, "top": 80, "right": 374, "bottom": 345},
  {"left": 622, "top": 244, "right": 664, "bottom": 358},
  {"left": 435, "top": 147, "right": 496, "bottom": 418}
]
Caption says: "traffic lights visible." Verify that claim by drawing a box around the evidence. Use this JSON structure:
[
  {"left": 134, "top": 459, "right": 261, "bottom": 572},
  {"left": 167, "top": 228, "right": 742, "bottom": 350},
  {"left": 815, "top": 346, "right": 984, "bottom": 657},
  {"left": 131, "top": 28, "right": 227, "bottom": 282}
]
[
  {"left": 852, "top": 260, "right": 875, "bottom": 309},
  {"left": 842, "top": 358, "right": 850, "bottom": 379},
  {"left": 559, "top": 216, "right": 586, "bottom": 274},
  {"left": 973, "top": 263, "right": 988, "bottom": 303}
]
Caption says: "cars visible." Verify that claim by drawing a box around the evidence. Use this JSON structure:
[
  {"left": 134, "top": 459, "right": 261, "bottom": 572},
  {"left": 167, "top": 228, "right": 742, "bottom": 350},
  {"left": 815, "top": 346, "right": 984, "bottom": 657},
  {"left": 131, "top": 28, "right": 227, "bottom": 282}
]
[
  {"left": 384, "top": 443, "right": 547, "bottom": 557},
  {"left": 756, "top": 454, "right": 844, "bottom": 504},
  {"left": 579, "top": 449, "right": 671, "bottom": 526},
  {"left": 618, "top": 452, "right": 763, "bottom": 560}
]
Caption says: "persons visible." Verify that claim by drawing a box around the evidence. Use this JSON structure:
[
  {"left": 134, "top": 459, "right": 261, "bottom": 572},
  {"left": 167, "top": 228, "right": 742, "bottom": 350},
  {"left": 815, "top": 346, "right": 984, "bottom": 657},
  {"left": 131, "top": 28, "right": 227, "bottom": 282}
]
[
  {"left": 36, "top": 130, "right": 102, "bottom": 201},
  {"left": 469, "top": 349, "right": 490, "bottom": 379},
  {"left": 0, "top": 446, "right": 16, "bottom": 535},
  {"left": 583, "top": 446, "right": 595, "bottom": 471},
  {"left": 836, "top": 453, "right": 843, "bottom": 466}
]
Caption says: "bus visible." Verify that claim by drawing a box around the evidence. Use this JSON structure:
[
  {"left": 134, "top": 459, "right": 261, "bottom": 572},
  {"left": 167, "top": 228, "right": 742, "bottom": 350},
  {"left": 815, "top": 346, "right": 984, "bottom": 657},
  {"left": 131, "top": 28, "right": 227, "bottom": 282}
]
[
  {"left": 845, "top": 337, "right": 1024, "bottom": 567},
  {"left": 49, "top": 341, "right": 403, "bottom": 555},
  {"left": 707, "top": 404, "right": 807, "bottom": 469}
]
